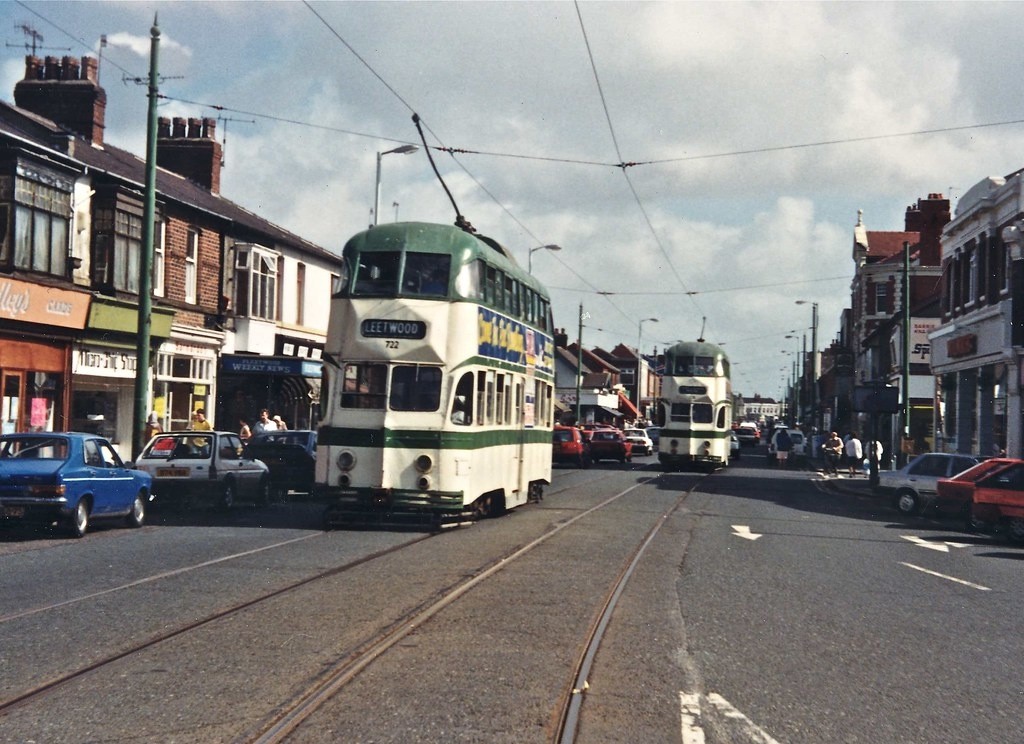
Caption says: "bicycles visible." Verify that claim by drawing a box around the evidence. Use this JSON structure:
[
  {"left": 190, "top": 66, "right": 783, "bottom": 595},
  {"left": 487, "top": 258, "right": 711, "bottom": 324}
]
[
  {"left": 821, "top": 446, "right": 837, "bottom": 475},
  {"left": 777, "top": 443, "right": 795, "bottom": 467}
]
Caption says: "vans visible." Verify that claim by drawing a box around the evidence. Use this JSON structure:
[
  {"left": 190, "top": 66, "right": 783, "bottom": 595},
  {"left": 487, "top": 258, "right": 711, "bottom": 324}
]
[{"left": 770, "top": 430, "right": 807, "bottom": 466}]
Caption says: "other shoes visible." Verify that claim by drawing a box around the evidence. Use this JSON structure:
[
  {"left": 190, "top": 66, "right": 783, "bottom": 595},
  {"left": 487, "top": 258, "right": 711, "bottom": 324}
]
[{"left": 849, "top": 472, "right": 853, "bottom": 478}]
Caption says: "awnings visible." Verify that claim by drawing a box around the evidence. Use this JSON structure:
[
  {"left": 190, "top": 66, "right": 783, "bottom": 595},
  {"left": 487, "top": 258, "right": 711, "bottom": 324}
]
[
  {"left": 618, "top": 392, "right": 644, "bottom": 417},
  {"left": 555, "top": 399, "right": 573, "bottom": 413},
  {"left": 600, "top": 406, "right": 625, "bottom": 417}
]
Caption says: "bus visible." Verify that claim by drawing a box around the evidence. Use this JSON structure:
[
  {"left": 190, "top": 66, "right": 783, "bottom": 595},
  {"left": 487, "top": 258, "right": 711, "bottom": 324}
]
[
  {"left": 651, "top": 343, "right": 735, "bottom": 472},
  {"left": 314, "top": 221, "right": 555, "bottom": 529}
]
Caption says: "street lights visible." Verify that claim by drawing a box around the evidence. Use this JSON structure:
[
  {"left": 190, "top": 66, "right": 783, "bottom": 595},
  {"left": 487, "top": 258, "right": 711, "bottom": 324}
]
[
  {"left": 778, "top": 299, "right": 819, "bottom": 431},
  {"left": 375, "top": 144, "right": 419, "bottom": 226},
  {"left": 528, "top": 243, "right": 563, "bottom": 274},
  {"left": 639, "top": 318, "right": 659, "bottom": 340}
]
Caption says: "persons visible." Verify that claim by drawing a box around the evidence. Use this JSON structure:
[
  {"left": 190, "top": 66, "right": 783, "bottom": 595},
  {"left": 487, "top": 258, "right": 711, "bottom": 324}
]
[
  {"left": 776, "top": 421, "right": 801, "bottom": 466},
  {"left": 624, "top": 418, "right": 653, "bottom": 429},
  {"left": 145, "top": 410, "right": 164, "bottom": 444},
  {"left": 554, "top": 327, "right": 568, "bottom": 346},
  {"left": 186, "top": 408, "right": 211, "bottom": 454},
  {"left": 676, "top": 363, "right": 714, "bottom": 377},
  {"left": 238, "top": 408, "right": 288, "bottom": 449},
  {"left": 821, "top": 430, "right": 884, "bottom": 478}
]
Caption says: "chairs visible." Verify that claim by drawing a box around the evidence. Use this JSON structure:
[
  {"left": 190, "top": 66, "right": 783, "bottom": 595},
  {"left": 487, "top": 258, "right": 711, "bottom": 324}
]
[
  {"left": 19, "top": 440, "right": 39, "bottom": 458},
  {"left": 174, "top": 442, "right": 191, "bottom": 456}
]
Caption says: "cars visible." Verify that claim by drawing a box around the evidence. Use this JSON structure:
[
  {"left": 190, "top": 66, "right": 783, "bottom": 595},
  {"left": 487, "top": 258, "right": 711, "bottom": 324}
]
[
  {"left": 874, "top": 452, "right": 1003, "bottom": 519},
  {"left": 580, "top": 426, "right": 660, "bottom": 463},
  {"left": 729, "top": 416, "right": 763, "bottom": 455},
  {"left": 239, "top": 429, "right": 318, "bottom": 495},
  {"left": 937, "top": 458, "right": 1024, "bottom": 546},
  {"left": 136, "top": 429, "right": 273, "bottom": 515},
  {"left": 0, "top": 431, "right": 154, "bottom": 540},
  {"left": 549, "top": 424, "right": 594, "bottom": 467}
]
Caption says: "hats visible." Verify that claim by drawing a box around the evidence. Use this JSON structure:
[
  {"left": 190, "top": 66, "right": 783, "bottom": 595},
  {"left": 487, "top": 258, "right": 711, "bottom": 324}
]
[{"left": 273, "top": 415, "right": 281, "bottom": 423}]
